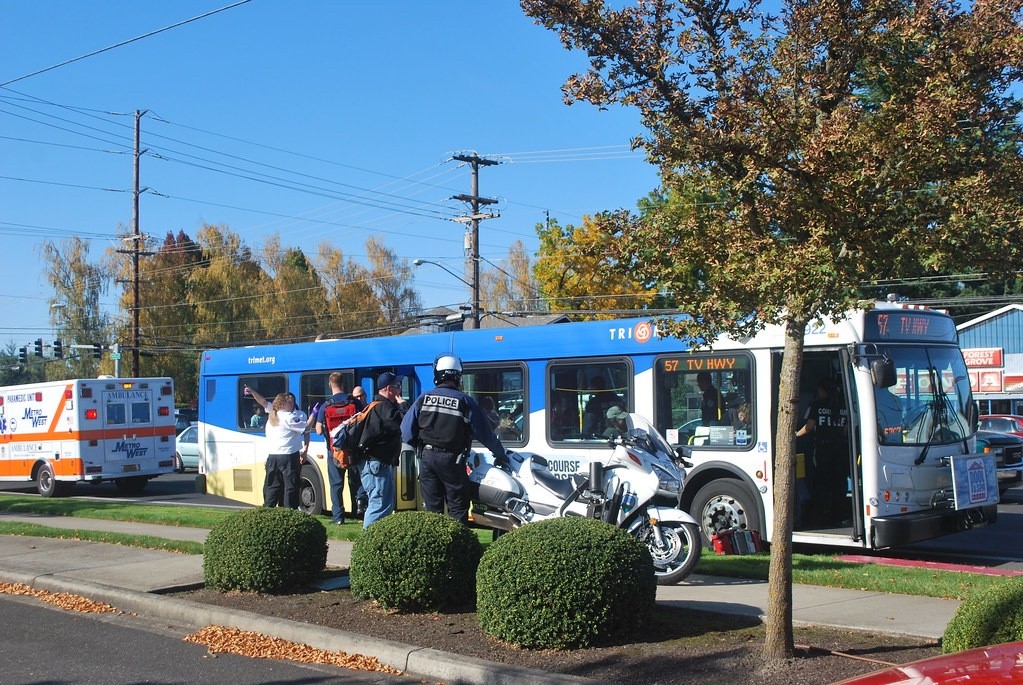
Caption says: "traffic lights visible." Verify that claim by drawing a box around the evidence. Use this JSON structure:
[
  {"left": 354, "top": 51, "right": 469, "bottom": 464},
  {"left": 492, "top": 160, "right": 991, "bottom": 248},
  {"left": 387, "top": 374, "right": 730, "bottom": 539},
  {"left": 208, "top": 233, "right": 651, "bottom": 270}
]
[
  {"left": 34, "top": 338, "right": 44, "bottom": 359},
  {"left": 53, "top": 340, "right": 63, "bottom": 361},
  {"left": 93, "top": 342, "right": 103, "bottom": 360},
  {"left": 18, "top": 348, "right": 27, "bottom": 363}
]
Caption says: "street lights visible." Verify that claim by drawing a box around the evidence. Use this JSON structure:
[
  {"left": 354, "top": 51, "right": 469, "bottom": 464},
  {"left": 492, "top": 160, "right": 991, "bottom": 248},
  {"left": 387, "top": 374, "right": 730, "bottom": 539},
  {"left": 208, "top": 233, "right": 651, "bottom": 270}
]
[
  {"left": 413, "top": 257, "right": 482, "bottom": 329},
  {"left": 51, "top": 303, "right": 141, "bottom": 378}
]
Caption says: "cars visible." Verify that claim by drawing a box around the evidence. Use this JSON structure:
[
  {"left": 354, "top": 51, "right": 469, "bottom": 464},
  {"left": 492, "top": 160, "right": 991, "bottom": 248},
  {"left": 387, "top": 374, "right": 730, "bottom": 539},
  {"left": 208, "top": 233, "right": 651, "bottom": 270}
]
[
  {"left": 175, "top": 407, "right": 198, "bottom": 436},
  {"left": 173, "top": 425, "right": 199, "bottom": 473},
  {"left": 976, "top": 413, "right": 1023, "bottom": 497}
]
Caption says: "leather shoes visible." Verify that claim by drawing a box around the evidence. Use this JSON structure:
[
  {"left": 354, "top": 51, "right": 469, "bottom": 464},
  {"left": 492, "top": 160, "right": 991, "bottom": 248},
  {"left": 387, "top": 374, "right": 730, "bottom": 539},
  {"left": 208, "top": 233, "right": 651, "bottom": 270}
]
[
  {"left": 329, "top": 520, "right": 342, "bottom": 525},
  {"left": 357, "top": 500, "right": 367, "bottom": 515}
]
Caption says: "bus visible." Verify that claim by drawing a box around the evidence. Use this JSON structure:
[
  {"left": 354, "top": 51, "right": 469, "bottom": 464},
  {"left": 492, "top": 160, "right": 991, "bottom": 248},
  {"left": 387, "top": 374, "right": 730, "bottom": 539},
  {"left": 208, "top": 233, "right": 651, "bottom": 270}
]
[{"left": 198, "top": 291, "right": 1001, "bottom": 554}]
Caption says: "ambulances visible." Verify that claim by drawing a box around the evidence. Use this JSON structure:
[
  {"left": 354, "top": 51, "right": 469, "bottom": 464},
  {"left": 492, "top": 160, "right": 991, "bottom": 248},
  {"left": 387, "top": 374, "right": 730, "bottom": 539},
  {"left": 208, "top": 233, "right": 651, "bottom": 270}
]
[{"left": 0, "top": 374, "right": 177, "bottom": 499}]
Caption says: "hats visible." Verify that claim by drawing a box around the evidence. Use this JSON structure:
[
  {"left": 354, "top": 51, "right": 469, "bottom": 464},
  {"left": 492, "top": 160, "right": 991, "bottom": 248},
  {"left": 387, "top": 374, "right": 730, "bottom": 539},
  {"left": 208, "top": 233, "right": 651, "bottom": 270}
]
[
  {"left": 377, "top": 372, "right": 404, "bottom": 389},
  {"left": 607, "top": 405, "right": 628, "bottom": 419}
]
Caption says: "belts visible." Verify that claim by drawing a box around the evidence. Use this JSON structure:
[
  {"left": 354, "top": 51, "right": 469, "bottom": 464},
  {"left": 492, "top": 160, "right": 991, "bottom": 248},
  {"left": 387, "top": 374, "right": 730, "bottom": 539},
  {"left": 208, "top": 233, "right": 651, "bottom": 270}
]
[{"left": 424, "top": 443, "right": 460, "bottom": 452}]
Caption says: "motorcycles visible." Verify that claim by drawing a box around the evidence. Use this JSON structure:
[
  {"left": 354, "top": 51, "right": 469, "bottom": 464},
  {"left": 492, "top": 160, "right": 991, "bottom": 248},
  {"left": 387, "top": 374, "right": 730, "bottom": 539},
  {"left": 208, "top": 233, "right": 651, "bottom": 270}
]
[{"left": 465, "top": 401, "right": 702, "bottom": 585}]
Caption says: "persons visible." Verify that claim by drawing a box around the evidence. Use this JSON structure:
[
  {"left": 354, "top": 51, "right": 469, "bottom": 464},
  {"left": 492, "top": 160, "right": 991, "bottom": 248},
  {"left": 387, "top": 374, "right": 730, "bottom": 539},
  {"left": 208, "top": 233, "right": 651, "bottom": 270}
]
[
  {"left": 401, "top": 352, "right": 509, "bottom": 526},
  {"left": 361, "top": 372, "right": 411, "bottom": 529},
  {"left": 717, "top": 402, "right": 752, "bottom": 436},
  {"left": 479, "top": 396, "right": 523, "bottom": 442},
  {"left": 582, "top": 377, "right": 627, "bottom": 434},
  {"left": 697, "top": 370, "right": 726, "bottom": 426},
  {"left": 602, "top": 406, "right": 628, "bottom": 438},
  {"left": 795, "top": 376, "right": 852, "bottom": 531},
  {"left": 244, "top": 383, "right": 320, "bottom": 508},
  {"left": 315, "top": 372, "right": 368, "bottom": 524}
]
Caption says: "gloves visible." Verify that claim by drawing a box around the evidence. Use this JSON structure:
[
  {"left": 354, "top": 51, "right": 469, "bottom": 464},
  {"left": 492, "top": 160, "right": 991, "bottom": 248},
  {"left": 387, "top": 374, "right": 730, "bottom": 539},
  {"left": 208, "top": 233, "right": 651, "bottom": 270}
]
[{"left": 494, "top": 454, "right": 510, "bottom": 467}]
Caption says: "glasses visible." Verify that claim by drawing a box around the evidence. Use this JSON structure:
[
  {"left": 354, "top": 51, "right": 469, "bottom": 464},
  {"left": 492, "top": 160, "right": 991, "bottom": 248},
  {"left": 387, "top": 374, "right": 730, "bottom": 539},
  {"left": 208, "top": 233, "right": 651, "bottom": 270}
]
[{"left": 389, "top": 383, "right": 402, "bottom": 390}]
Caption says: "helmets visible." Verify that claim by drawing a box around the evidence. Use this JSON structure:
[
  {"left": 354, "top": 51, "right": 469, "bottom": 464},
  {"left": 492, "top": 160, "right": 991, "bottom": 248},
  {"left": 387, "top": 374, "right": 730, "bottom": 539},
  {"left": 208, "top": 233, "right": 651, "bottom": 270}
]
[{"left": 434, "top": 356, "right": 463, "bottom": 384}]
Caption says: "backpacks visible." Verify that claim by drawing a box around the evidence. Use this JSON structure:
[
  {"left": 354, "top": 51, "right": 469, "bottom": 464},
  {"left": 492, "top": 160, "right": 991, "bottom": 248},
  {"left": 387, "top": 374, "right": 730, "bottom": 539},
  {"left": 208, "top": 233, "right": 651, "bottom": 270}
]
[
  {"left": 324, "top": 397, "right": 359, "bottom": 452},
  {"left": 331, "top": 401, "right": 386, "bottom": 451}
]
